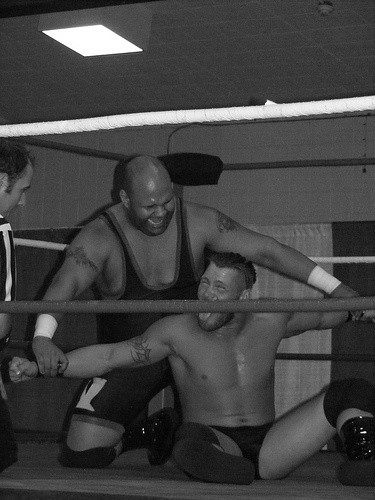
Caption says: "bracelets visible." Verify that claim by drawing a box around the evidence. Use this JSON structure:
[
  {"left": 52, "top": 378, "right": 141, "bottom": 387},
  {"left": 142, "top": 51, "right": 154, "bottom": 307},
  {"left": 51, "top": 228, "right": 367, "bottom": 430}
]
[
  {"left": 347, "top": 309, "right": 365, "bottom": 323},
  {"left": 56, "top": 360, "right": 65, "bottom": 379}
]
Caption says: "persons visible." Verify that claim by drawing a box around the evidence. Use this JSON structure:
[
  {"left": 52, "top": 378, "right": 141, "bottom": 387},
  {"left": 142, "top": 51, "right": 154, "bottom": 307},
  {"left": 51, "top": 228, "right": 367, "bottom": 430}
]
[
  {"left": 9, "top": 253, "right": 375, "bottom": 487},
  {"left": 31, "top": 153, "right": 375, "bottom": 468},
  {"left": 0, "top": 138, "right": 36, "bottom": 471}
]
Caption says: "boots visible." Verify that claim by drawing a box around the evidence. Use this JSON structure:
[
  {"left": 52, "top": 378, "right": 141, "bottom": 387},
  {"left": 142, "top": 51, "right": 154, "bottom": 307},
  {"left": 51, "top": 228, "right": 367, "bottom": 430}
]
[
  {"left": 337, "top": 416, "right": 375, "bottom": 487},
  {"left": 122, "top": 406, "right": 176, "bottom": 466},
  {"left": 172, "top": 438, "right": 254, "bottom": 484}
]
